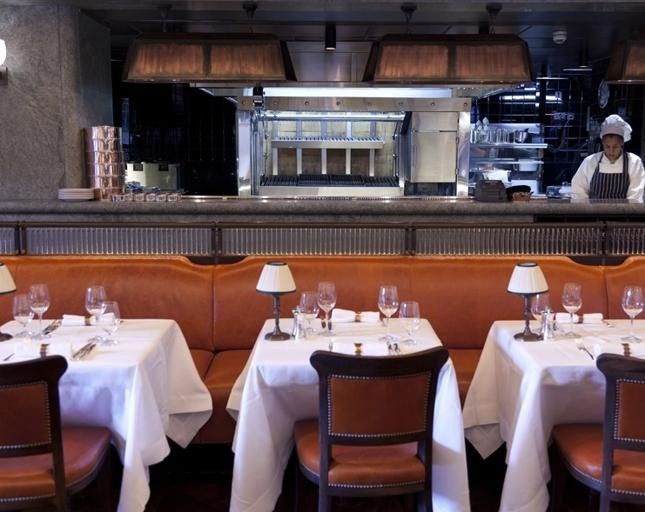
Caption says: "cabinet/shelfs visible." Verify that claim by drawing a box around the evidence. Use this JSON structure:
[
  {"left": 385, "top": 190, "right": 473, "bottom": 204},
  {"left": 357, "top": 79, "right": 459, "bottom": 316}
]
[{"left": 470, "top": 124, "right": 548, "bottom": 189}]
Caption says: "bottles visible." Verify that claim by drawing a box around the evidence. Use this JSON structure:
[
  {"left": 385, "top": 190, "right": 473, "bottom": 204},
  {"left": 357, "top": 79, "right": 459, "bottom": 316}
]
[{"left": 537, "top": 306, "right": 555, "bottom": 340}]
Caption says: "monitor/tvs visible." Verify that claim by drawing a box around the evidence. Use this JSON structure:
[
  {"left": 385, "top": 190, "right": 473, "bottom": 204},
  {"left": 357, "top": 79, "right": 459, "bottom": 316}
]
[{"left": 404, "top": 180, "right": 456, "bottom": 196}]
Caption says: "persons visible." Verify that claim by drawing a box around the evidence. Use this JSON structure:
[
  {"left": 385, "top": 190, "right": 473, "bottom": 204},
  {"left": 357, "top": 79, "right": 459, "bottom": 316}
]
[{"left": 569, "top": 113, "right": 645, "bottom": 205}]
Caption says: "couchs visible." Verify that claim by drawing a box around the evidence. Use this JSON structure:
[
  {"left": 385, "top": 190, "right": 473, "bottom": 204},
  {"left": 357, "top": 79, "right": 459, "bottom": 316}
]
[
  {"left": 0, "top": 255, "right": 214, "bottom": 444},
  {"left": 604, "top": 255, "right": 645, "bottom": 320},
  {"left": 193, "top": 255, "right": 609, "bottom": 443}
]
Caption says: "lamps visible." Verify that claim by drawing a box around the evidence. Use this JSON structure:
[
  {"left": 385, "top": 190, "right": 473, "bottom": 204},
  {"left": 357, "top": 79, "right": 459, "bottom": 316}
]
[
  {"left": 0, "top": 39, "right": 8, "bottom": 86},
  {"left": 507, "top": 262, "right": 549, "bottom": 341},
  {"left": 0, "top": 262, "right": 16, "bottom": 343},
  {"left": 256, "top": 262, "right": 296, "bottom": 341},
  {"left": 325, "top": 22, "right": 338, "bottom": 51},
  {"left": 552, "top": 25, "right": 566, "bottom": 44}
]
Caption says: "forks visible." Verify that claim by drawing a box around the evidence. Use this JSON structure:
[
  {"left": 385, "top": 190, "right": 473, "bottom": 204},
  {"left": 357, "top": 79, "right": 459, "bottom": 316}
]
[{"left": 575, "top": 340, "right": 596, "bottom": 362}]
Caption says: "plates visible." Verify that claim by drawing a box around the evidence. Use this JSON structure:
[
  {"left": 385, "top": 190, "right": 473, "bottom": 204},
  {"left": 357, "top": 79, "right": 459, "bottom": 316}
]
[{"left": 57, "top": 188, "right": 101, "bottom": 203}]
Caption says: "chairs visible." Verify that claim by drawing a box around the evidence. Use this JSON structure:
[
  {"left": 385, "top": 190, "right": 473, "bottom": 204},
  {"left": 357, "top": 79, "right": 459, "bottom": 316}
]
[
  {"left": 290, "top": 346, "right": 449, "bottom": 512},
  {"left": 548, "top": 355, "right": 644, "bottom": 512},
  {"left": 0, "top": 357, "right": 113, "bottom": 512}
]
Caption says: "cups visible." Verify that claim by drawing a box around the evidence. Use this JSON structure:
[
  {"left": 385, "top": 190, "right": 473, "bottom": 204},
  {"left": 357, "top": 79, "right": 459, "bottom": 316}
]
[
  {"left": 470, "top": 126, "right": 510, "bottom": 143},
  {"left": 512, "top": 130, "right": 529, "bottom": 143},
  {"left": 82, "top": 124, "right": 125, "bottom": 202}
]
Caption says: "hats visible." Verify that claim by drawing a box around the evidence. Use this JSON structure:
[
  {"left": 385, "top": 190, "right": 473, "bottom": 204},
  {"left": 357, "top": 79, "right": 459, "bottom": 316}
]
[{"left": 600, "top": 113, "right": 632, "bottom": 143}]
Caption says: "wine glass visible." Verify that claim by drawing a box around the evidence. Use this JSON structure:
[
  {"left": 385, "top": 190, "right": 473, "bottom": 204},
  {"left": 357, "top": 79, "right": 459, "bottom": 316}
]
[
  {"left": 290, "top": 280, "right": 337, "bottom": 339},
  {"left": 530, "top": 293, "right": 549, "bottom": 334},
  {"left": 619, "top": 284, "right": 643, "bottom": 343},
  {"left": 12, "top": 282, "right": 54, "bottom": 340},
  {"left": 81, "top": 284, "right": 121, "bottom": 347},
  {"left": 560, "top": 281, "right": 583, "bottom": 338},
  {"left": 377, "top": 284, "right": 420, "bottom": 346}
]
